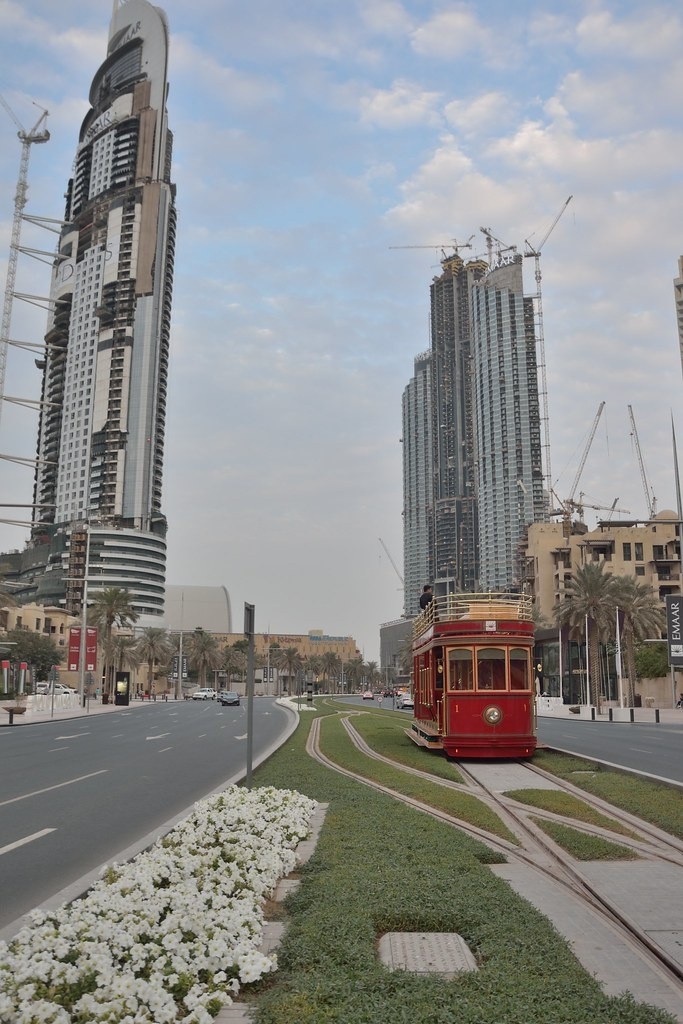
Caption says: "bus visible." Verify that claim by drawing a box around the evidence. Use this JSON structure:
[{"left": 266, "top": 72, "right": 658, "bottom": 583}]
[{"left": 403, "top": 589, "right": 542, "bottom": 758}]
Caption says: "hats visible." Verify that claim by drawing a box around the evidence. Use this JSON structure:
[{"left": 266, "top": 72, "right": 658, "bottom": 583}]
[{"left": 424, "top": 585, "right": 431, "bottom": 592}]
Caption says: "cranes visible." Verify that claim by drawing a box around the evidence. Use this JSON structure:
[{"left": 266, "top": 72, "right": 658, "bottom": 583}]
[
  {"left": 552, "top": 400, "right": 606, "bottom": 539},
  {"left": 389, "top": 235, "right": 476, "bottom": 254},
  {"left": 628, "top": 404, "right": 657, "bottom": 520},
  {"left": 0, "top": 93, "right": 49, "bottom": 405},
  {"left": 563, "top": 492, "right": 630, "bottom": 523},
  {"left": 523, "top": 195, "right": 574, "bottom": 512},
  {"left": 466, "top": 238, "right": 516, "bottom": 262},
  {"left": 596, "top": 498, "right": 620, "bottom": 521},
  {"left": 378, "top": 538, "right": 404, "bottom": 585}
]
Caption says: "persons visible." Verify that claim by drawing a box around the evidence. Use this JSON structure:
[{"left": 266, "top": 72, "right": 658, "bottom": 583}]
[
  {"left": 420, "top": 585, "right": 439, "bottom": 622},
  {"left": 137, "top": 688, "right": 145, "bottom": 699},
  {"left": 677, "top": 693, "right": 683, "bottom": 708},
  {"left": 96, "top": 688, "right": 99, "bottom": 699}
]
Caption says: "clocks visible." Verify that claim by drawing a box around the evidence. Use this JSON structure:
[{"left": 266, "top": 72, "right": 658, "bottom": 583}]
[{"left": 486, "top": 620, "right": 496, "bottom": 632}]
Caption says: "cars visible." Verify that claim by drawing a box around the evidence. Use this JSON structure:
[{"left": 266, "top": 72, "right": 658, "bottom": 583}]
[
  {"left": 363, "top": 691, "right": 374, "bottom": 700},
  {"left": 37, "top": 683, "right": 49, "bottom": 694},
  {"left": 217, "top": 691, "right": 226, "bottom": 702},
  {"left": 193, "top": 688, "right": 217, "bottom": 700},
  {"left": 55, "top": 684, "right": 78, "bottom": 694},
  {"left": 396, "top": 694, "right": 414, "bottom": 709},
  {"left": 221, "top": 692, "right": 240, "bottom": 706},
  {"left": 383, "top": 690, "right": 409, "bottom": 698}
]
